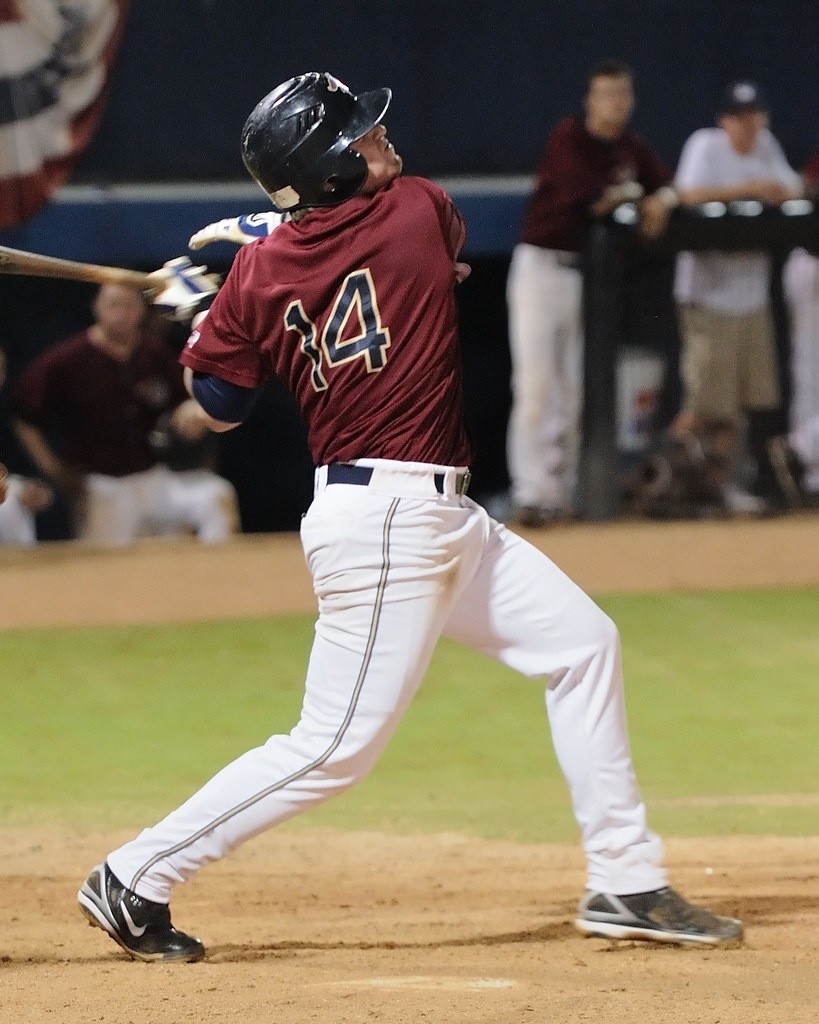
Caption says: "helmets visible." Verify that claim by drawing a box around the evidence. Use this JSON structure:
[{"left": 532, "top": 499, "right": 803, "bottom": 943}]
[{"left": 241, "top": 72, "right": 392, "bottom": 213}]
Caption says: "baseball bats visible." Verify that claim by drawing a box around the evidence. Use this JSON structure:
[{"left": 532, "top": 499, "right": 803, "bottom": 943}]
[{"left": 0, "top": 246, "right": 221, "bottom": 294}]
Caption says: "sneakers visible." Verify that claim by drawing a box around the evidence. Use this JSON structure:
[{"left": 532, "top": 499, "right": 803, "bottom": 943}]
[
  {"left": 77, "top": 860, "right": 205, "bottom": 963},
  {"left": 575, "top": 886, "right": 744, "bottom": 946}
]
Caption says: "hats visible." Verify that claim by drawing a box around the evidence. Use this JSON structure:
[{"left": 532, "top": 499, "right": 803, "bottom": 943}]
[{"left": 725, "top": 79, "right": 766, "bottom": 111}]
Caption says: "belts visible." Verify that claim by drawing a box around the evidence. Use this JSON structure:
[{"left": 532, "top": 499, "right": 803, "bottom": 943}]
[{"left": 327, "top": 463, "right": 471, "bottom": 496}]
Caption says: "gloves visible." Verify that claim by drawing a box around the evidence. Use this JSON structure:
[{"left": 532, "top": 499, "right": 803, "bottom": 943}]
[
  {"left": 188, "top": 211, "right": 293, "bottom": 251},
  {"left": 143, "top": 255, "right": 222, "bottom": 331}
]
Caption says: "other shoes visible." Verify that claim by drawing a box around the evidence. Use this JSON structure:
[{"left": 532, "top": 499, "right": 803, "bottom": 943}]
[{"left": 518, "top": 507, "right": 553, "bottom": 528}]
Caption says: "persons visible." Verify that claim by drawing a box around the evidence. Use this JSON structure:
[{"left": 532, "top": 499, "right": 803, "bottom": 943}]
[
  {"left": 77, "top": 70, "right": 751, "bottom": 965},
  {"left": 2, "top": 66, "right": 819, "bottom": 543}
]
